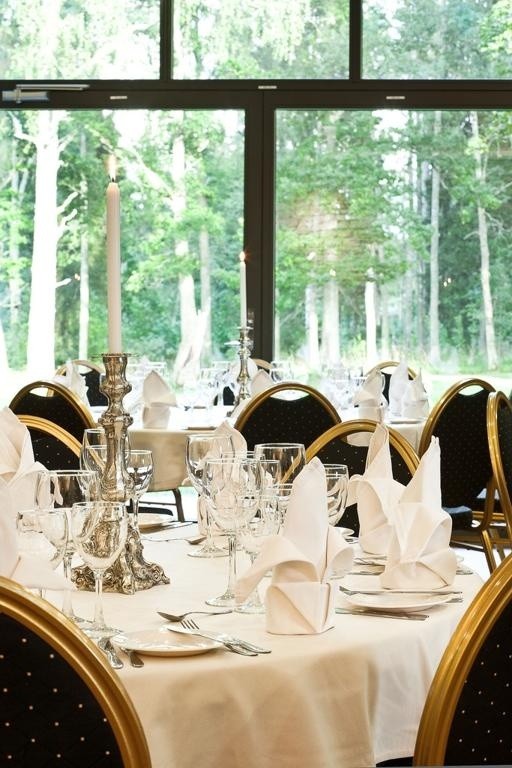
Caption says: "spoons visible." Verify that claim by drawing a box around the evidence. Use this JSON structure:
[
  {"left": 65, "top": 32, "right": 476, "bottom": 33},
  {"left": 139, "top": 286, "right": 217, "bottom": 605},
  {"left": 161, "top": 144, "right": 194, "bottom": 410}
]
[
  {"left": 140, "top": 537, "right": 207, "bottom": 546},
  {"left": 156, "top": 610, "right": 233, "bottom": 622}
]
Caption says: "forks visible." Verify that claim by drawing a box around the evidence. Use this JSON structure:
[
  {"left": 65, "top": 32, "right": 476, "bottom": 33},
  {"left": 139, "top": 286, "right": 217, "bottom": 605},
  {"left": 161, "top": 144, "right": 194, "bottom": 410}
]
[{"left": 179, "top": 619, "right": 272, "bottom": 653}]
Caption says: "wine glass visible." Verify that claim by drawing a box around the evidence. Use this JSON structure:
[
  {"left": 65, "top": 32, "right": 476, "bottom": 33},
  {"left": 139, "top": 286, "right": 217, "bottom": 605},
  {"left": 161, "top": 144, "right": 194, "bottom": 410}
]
[
  {"left": 61, "top": 357, "right": 426, "bottom": 431},
  {"left": 34, "top": 469, "right": 103, "bottom": 631},
  {"left": 200, "top": 459, "right": 261, "bottom": 609},
  {"left": 185, "top": 434, "right": 237, "bottom": 561},
  {"left": 71, "top": 501, "right": 128, "bottom": 638},
  {"left": 126, "top": 449, "right": 155, "bottom": 528}
]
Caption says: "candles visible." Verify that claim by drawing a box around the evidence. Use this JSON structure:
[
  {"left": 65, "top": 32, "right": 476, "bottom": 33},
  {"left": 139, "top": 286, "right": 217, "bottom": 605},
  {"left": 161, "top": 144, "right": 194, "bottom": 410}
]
[
  {"left": 105, "top": 154, "right": 122, "bottom": 353},
  {"left": 238, "top": 251, "right": 249, "bottom": 327}
]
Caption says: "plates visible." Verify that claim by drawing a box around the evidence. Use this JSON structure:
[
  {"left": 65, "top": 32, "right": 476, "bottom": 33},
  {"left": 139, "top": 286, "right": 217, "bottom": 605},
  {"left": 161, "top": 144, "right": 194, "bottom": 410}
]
[
  {"left": 113, "top": 629, "right": 229, "bottom": 657},
  {"left": 349, "top": 590, "right": 456, "bottom": 612}
]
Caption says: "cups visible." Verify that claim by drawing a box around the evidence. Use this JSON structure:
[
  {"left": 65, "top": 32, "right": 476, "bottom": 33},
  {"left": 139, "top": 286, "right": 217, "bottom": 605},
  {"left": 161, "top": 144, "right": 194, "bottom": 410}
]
[
  {"left": 234, "top": 500, "right": 282, "bottom": 568},
  {"left": 17, "top": 511, "right": 69, "bottom": 599},
  {"left": 80, "top": 429, "right": 132, "bottom": 501},
  {"left": 80, "top": 445, "right": 107, "bottom": 475},
  {"left": 240, "top": 457, "right": 282, "bottom": 500},
  {"left": 264, "top": 482, "right": 294, "bottom": 532},
  {"left": 322, "top": 463, "right": 351, "bottom": 527},
  {"left": 253, "top": 441, "right": 306, "bottom": 513}
]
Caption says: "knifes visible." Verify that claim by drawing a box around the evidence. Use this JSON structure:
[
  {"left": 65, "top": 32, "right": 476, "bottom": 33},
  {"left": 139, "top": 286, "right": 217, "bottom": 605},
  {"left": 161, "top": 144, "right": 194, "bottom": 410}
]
[
  {"left": 339, "top": 586, "right": 463, "bottom": 595},
  {"left": 165, "top": 625, "right": 258, "bottom": 657},
  {"left": 99, "top": 637, "right": 123, "bottom": 670}
]
